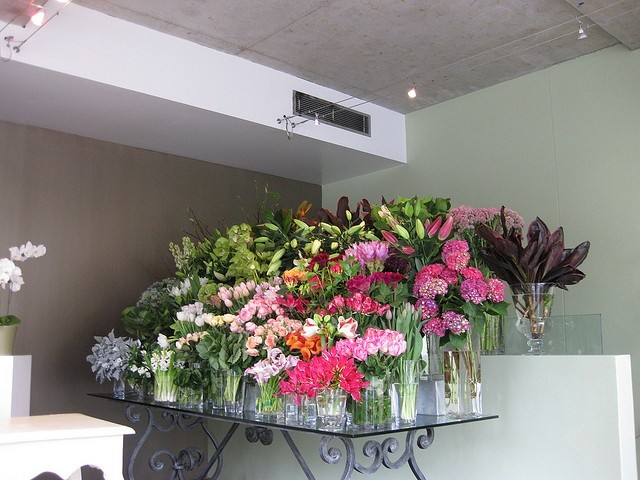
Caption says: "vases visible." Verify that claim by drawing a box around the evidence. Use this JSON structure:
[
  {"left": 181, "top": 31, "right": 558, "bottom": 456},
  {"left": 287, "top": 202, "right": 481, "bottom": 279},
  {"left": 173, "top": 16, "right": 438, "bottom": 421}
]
[
  {"left": 105, "top": 351, "right": 484, "bottom": 430},
  {"left": 477, "top": 311, "right": 505, "bottom": 354}
]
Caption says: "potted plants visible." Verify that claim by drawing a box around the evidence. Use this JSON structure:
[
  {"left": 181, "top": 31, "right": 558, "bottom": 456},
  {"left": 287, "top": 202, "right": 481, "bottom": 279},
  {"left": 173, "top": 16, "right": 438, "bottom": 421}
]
[
  {"left": 0, "top": 242, "right": 50, "bottom": 355},
  {"left": 473, "top": 213, "right": 590, "bottom": 355}
]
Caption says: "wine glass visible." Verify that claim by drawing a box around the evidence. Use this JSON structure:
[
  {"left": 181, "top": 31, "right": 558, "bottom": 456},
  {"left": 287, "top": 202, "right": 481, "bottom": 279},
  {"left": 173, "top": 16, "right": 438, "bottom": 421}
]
[{"left": 507, "top": 281, "right": 557, "bottom": 356}]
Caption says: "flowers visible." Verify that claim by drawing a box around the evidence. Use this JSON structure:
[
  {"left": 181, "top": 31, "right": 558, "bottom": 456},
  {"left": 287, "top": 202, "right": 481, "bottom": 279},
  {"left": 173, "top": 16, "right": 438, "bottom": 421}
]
[{"left": 87, "top": 185, "right": 525, "bottom": 413}]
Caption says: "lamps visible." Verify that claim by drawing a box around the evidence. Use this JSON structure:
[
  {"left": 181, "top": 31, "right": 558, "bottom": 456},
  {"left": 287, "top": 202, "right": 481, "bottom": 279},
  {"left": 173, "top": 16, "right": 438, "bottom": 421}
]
[
  {"left": 405, "top": 81, "right": 421, "bottom": 101},
  {"left": 24, "top": 1, "right": 45, "bottom": 28},
  {"left": 575, "top": 17, "right": 588, "bottom": 40}
]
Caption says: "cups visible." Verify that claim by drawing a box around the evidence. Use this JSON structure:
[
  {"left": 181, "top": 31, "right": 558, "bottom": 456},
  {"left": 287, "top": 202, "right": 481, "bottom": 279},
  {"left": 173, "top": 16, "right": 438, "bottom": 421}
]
[
  {"left": 253, "top": 373, "right": 382, "bottom": 434},
  {"left": 222, "top": 373, "right": 245, "bottom": 417},
  {"left": 212, "top": 373, "right": 228, "bottom": 409},
  {"left": 110, "top": 372, "right": 154, "bottom": 402},
  {"left": 444, "top": 349, "right": 482, "bottom": 418},
  {"left": 180, "top": 380, "right": 203, "bottom": 416},
  {"left": 389, "top": 360, "right": 420, "bottom": 428}
]
